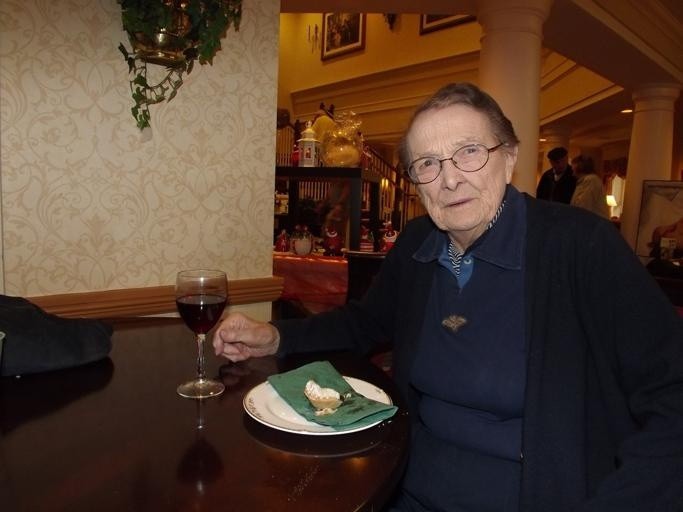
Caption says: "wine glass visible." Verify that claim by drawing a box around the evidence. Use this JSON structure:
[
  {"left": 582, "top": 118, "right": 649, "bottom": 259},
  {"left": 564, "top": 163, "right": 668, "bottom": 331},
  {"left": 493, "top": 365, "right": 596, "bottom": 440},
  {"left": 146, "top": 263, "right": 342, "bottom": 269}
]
[
  {"left": 174, "top": 268, "right": 228, "bottom": 398},
  {"left": 179, "top": 399, "right": 225, "bottom": 492}
]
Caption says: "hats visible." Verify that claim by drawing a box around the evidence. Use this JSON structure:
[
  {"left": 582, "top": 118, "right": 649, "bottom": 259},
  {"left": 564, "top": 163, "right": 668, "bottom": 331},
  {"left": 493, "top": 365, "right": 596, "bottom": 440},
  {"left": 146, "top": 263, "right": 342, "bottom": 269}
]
[{"left": 547, "top": 147, "right": 568, "bottom": 161}]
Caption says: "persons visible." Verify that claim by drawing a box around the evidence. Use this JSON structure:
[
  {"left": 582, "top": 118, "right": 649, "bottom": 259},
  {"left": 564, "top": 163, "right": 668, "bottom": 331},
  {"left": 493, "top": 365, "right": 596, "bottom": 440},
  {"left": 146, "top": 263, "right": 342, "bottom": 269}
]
[
  {"left": 213, "top": 80, "right": 683, "bottom": 510},
  {"left": 536, "top": 147, "right": 582, "bottom": 208},
  {"left": 568, "top": 154, "right": 612, "bottom": 221},
  {"left": 324, "top": 12, "right": 362, "bottom": 52}
]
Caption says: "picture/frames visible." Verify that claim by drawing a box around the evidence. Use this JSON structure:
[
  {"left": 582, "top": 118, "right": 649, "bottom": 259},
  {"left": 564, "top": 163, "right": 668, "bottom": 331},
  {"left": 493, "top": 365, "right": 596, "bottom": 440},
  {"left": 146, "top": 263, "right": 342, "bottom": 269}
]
[
  {"left": 320, "top": 11, "right": 366, "bottom": 62},
  {"left": 419, "top": 12, "right": 476, "bottom": 35}
]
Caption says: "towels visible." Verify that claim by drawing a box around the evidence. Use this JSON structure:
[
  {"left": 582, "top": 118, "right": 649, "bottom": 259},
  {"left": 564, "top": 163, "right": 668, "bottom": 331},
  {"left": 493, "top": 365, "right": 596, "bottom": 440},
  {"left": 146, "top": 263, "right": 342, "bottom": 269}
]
[{"left": 268, "top": 360, "right": 398, "bottom": 432}]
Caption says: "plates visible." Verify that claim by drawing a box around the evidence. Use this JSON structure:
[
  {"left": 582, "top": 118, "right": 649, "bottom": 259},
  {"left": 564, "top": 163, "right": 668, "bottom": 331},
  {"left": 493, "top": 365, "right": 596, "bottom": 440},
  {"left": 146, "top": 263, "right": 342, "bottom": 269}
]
[
  {"left": 244, "top": 375, "right": 395, "bottom": 436},
  {"left": 244, "top": 418, "right": 391, "bottom": 458}
]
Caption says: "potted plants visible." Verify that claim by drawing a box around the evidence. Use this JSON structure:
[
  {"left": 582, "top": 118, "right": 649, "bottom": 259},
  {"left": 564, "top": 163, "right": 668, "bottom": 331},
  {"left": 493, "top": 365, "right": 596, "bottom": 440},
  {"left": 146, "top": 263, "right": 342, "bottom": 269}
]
[{"left": 118, "top": 0, "right": 242, "bottom": 132}]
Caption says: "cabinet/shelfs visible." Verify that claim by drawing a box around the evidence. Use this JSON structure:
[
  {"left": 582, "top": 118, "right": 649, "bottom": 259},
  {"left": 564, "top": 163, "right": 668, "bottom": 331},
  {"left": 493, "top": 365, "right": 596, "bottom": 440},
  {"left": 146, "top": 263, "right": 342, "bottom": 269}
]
[{"left": 272, "top": 104, "right": 426, "bottom": 315}]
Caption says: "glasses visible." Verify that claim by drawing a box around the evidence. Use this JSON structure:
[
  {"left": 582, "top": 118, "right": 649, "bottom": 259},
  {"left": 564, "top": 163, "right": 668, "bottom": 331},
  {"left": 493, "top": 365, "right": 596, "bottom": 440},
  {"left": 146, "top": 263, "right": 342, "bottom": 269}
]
[{"left": 404, "top": 142, "right": 503, "bottom": 185}]
